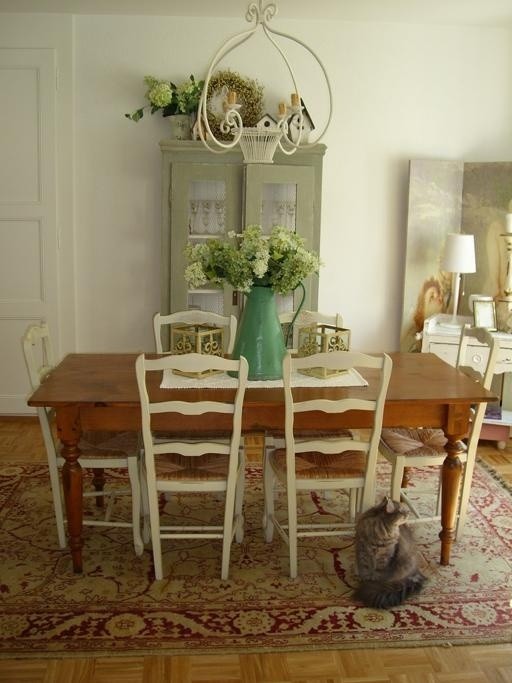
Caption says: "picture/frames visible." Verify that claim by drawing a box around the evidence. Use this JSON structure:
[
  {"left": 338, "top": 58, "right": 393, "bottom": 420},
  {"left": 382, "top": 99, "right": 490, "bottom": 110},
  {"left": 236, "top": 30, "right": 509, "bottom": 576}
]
[{"left": 470, "top": 298, "right": 498, "bottom": 333}]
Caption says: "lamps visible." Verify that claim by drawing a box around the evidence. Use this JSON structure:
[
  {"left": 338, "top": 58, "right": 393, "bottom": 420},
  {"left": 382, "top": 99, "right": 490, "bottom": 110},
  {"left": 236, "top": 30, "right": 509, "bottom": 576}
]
[
  {"left": 439, "top": 226, "right": 478, "bottom": 330},
  {"left": 195, "top": 0, "right": 337, "bottom": 166}
]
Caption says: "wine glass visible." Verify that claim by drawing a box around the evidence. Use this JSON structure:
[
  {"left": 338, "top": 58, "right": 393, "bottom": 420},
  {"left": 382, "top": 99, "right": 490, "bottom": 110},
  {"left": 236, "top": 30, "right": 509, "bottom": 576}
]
[
  {"left": 262, "top": 196, "right": 296, "bottom": 234},
  {"left": 188, "top": 199, "right": 225, "bottom": 235}
]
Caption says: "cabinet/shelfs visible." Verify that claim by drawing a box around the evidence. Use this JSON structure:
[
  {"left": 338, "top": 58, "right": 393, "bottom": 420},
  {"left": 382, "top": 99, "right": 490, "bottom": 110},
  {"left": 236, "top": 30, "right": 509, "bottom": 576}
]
[
  {"left": 156, "top": 135, "right": 328, "bottom": 351},
  {"left": 420, "top": 309, "right": 512, "bottom": 452}
]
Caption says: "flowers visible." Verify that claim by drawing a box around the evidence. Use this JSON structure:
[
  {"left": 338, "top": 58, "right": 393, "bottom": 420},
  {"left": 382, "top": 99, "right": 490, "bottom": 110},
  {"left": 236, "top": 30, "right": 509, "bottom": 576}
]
[
  {"left": 178, "top": 218, "right": 324, "bottom": 308},
  {"left": 198, "top": 68, "right": 265, "bottom": 141},
  {"left": 123, "top": 69, "right": 207, "bottom": 125}
]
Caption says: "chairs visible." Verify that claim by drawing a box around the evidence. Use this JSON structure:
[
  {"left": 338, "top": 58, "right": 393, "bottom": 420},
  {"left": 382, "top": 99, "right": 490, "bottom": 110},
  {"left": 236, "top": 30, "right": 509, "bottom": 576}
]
[
  {"left": 141, "top": 306, "right": 246, "bottom": 450},
  {"left": 131, "top": 346, "right": 247, "bottom": 583},
  {"left": 260, "top": 342, "right": 395, "bottom": 578},
  {"left": 347, "top": 319, "right": 501, "bottom": 542},
  {"left": 261, "top": 307, "right": 361, "bottom": 495},
  {"left": 21, "top": 319, "right": 153, "bottom": 558}
]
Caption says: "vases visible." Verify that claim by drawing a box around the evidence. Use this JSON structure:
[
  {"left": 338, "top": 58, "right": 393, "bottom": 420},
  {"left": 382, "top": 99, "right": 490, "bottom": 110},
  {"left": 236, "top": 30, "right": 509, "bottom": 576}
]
[
  {"left": 222, "top": 275, "right": 313, "bottom": 384},
  {"left": 160, "top": 110, "right": 194, "bottom": 142}
]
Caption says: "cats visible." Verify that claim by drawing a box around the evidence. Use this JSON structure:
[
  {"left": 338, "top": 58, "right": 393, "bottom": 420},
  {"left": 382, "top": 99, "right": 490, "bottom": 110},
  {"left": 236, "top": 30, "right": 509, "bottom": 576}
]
[{"left": 352, "top": 495, "right": 428, "bottom": 609}]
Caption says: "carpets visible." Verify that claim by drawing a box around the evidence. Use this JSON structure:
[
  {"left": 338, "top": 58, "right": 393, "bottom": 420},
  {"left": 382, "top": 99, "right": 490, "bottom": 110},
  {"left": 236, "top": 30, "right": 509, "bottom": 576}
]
[{"left": 1, "top": 439, "right": 511, "bottom": 662}]
